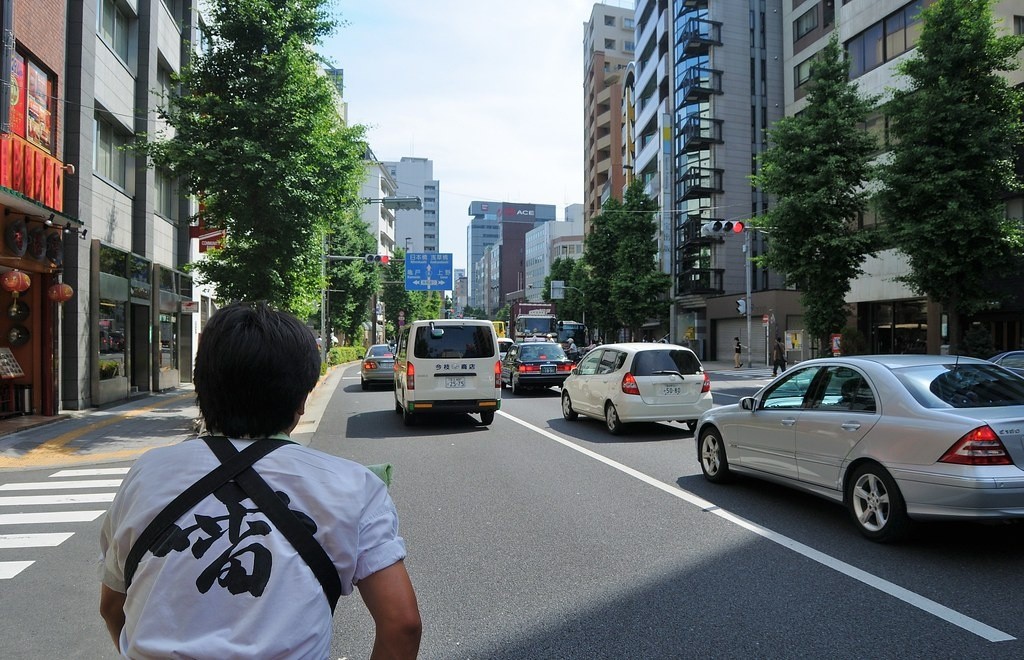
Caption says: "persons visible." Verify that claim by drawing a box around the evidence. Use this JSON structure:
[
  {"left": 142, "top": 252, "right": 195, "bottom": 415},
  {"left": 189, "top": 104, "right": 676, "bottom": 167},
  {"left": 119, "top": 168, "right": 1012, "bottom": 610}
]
[
  {"left": 531, "top": 326, "right": 695, "bottom": 364},
  {"left": 734, "top": 337, "right": 743, "bottom": 368},
  {"left": 772, "top": 337, "right": 786, "bottom": 377},
  {"left": 463, "top": 344, "right": 476, "bottom": 358},
  {"left": 98, "top": 302, "right": 423, "bottom": 660}
]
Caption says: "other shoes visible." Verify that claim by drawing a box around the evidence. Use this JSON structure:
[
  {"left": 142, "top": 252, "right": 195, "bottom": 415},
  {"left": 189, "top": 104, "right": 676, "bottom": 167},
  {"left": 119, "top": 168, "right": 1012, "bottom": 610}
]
[
  {"left": 740, "top": 364, "right": 743, "bottom": 367},
  {"left": 734, "top": 366, "right": 740, "bottom": 368},
  {"left": 769, "top": 373, "right": 778, "bottom": 377}
]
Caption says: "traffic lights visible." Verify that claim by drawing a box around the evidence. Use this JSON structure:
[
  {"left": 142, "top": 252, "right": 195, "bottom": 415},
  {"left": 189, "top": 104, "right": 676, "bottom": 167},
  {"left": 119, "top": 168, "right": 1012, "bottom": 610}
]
[
  {"left": 735, "top": 299, "right": 746, "bottom": 315},
  {"left": 708, "top": 220, "right": 745, "bottom": 235},
  {"left": 365, "top": 253, "right": 390, "bottom": 264}
]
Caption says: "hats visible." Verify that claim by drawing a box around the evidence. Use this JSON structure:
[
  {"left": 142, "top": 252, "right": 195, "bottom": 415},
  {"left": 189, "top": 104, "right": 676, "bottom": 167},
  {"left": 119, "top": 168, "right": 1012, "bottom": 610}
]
[{"left": 547, "top": 334, "right": 552, "bottom": 337}]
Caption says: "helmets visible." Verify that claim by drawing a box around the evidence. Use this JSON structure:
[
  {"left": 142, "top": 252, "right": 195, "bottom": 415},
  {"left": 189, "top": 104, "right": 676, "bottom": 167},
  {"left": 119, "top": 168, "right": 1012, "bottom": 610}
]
[{"left": 566, "top": 338, "right": 574, "bottom": 344}]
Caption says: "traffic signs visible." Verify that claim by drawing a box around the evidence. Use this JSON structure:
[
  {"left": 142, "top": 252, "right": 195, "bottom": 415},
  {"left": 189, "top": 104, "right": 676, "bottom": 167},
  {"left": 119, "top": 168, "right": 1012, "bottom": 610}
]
[{"left": 405, "top": 253, "right": 454, "bottom": 292}]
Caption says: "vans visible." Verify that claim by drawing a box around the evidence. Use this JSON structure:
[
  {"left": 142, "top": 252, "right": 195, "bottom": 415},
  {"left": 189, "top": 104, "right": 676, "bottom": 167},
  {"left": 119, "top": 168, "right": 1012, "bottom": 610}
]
[{"left": 392, "top": 317, "right": 502, "bottom": 427}]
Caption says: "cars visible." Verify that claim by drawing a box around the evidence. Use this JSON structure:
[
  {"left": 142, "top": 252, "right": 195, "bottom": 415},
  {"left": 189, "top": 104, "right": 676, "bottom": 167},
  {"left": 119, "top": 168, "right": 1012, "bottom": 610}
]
[
  {"left": 695, "top": 354, "right": 1024, "bottom": 546},
  {"left": 561, "top": 343, "right": 713, "bottom": 436},
  {"left": 500, "top": 341, "right": 577, "bottom": 396},
  {"left": 497, "top": 337, "right": 514, "bottom": 359},
  {"left": 964, "top": 351, "right": 1024, "bottom": 392},
  {"left": 358, "top": 344, "right": 396, "bottom": 391}
]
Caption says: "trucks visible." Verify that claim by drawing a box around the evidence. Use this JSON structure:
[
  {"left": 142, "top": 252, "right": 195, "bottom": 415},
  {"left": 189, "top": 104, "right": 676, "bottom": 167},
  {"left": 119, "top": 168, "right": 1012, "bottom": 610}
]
[{"left": 509, "top": 300, "right": 563, "bottom": 344}]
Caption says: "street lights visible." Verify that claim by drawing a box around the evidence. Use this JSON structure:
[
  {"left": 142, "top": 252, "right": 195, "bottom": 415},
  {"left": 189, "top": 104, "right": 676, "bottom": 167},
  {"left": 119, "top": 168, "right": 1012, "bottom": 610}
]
[{"left": 406, "top": 237, "right": 412, "bottom": 252}]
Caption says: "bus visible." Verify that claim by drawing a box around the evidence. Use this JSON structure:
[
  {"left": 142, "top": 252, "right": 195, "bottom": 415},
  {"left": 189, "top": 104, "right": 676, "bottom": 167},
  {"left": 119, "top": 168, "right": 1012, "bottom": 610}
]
[{"left": 490, "top": 312, "right": 595, "bottom": 365}]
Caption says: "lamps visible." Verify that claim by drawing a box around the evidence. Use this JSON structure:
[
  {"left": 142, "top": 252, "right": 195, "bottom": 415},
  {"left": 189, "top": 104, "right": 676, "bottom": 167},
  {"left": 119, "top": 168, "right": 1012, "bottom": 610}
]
[
  {"left": 4, "top": 208, "right": 55, "bottom": 227},
  {"left": 60, "top": 164, "right": 75, "bottom": 175},
  {"left": 53, "top": 227, "right": 87, "bottom": 239},
  {"left": 26, "top": 217, "right": 72, "bottom": 234}
]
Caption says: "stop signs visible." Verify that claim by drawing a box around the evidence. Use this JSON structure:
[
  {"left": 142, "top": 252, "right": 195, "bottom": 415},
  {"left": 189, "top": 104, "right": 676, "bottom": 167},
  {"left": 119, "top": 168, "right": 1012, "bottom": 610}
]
[{"left": 762, "top": 314, "right": 769, "bottom": 324}]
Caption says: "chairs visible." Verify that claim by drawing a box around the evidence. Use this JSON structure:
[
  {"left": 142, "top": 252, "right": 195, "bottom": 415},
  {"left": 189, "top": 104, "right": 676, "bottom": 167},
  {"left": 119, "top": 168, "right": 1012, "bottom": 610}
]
[{"left": 837, "top": 376, "right": 869, "bottom": 408}]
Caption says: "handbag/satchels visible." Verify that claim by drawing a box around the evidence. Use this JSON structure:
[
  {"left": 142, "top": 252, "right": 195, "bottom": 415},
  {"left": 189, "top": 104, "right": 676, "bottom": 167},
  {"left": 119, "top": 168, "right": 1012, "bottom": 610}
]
[{"left": 782, "top": 353, "right": 788, "bottom": 362}]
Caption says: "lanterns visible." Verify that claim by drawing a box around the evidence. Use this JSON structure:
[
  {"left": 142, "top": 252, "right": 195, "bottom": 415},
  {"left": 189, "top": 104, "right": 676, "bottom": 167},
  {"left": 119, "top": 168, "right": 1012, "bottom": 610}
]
[
  {"left": 0, "top": 269, "right": 30, "bottom": 297},
  {"left": 47, "top": 282, "right": 74, "bottom": 303}
]
